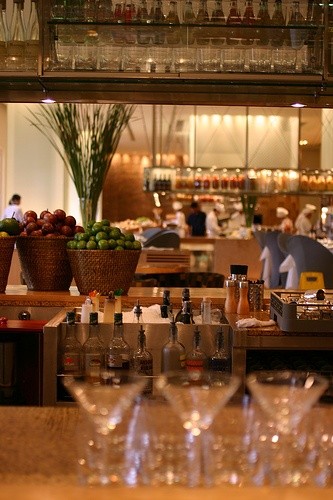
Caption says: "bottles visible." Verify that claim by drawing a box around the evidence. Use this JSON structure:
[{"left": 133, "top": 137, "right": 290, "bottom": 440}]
[
  {"left": 186, "top": 330, "right": 208, "bottom": 387},
  {"left": 143, "top": 164, "right": 333, "bottom": 193},
  {"left": 281, "top": 288, "right": 333, "bottom": 320},
  {"left": 208, "top": 327, "right": 232, "bottom": 386},
  {"left": 161, "top": 322, "right": 186, "bottom": 384},
  {"left": 132, "top": 325, "right": 154, "bottom": 395},
  {"left": 106, "top": 312, "right": 132, "bottom": 385},
  {"left": 224, "top": 274, "right": 266, "bottom": 315},
  {"left": 61, "top": 312, "right": 82, "bottom": 380},
  {"left": 81, "top": 289, "right": 211, "bottom": 324},
  {"left": 83, "top": 312, "right": 106, "bottom": 385}
]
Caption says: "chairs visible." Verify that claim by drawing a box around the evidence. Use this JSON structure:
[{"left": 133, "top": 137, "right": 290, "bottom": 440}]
[
  {"left": 250, "top": 229, "right": 333, "bottom": 290},
  {"left": 141, "top": 226, "right": 181, "bottom": 251}
]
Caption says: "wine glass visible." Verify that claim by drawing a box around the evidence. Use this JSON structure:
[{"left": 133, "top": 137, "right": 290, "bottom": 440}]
[{"left": 60, "top": 370, "right": 333, "bottom": 487}]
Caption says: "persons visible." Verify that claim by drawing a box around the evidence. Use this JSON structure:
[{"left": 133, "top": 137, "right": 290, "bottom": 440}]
[
  {"left": 276, "top": 204, "right": 318, "bottom": 238},
  {"left": 3, "top": 195, "right": 24, "bottom": 223},
  {"left": 171, "top": 202, "right": 246, "bottom": 238}
]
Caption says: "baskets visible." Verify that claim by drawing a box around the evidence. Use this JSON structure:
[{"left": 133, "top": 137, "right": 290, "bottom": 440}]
[
  {"left": 0, "top": 236, "right": 15, "bottom": 293},
  {"left": 67, "top": 248, "right": 142, "bottom": 296},
  {"left": 15, "top": 236, "right": 75, "bottom": 291}
]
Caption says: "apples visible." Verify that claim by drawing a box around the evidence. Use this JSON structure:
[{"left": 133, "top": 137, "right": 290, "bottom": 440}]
[
  {"left": 66, "top": 219, "right": 141, "bottom": 250},
  {"left": 0, "top": 208, "right": 85, "bottom": 237}
]
